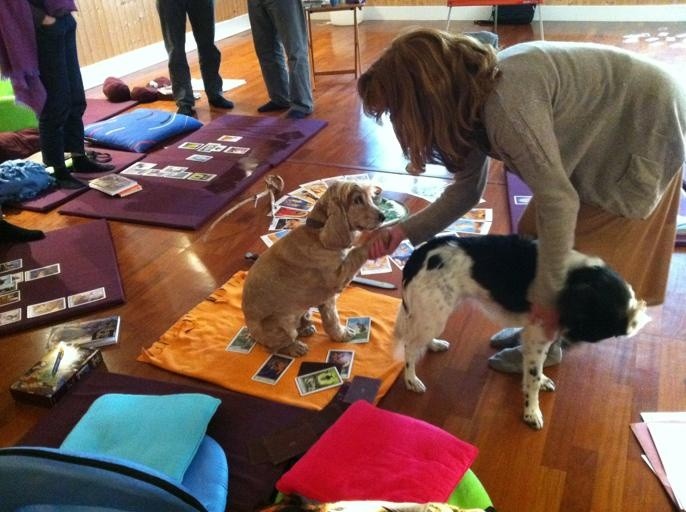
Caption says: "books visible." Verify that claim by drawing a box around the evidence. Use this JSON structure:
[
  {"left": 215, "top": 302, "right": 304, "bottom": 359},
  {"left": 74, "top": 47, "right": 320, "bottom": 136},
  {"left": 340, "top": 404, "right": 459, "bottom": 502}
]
[
  {"left": 9, "top": 341, "right": 103, "bottom": 409},
  {"left": 88, "top": 173, "right": 138, "bottom": 196},
  {"left": 44, "top": 315, "right": 121, "bottom": 352},
  {"left": 117, "top": 184, "right": 143, "bottom": 197}
]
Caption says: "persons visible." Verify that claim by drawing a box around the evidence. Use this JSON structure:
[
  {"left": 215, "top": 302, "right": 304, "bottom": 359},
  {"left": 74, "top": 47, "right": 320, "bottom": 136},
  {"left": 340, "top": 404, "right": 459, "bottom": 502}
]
[
  {"left": 1, "top": 0, "right": 116, "bottom": 189},
  {"left": 247, "top": 0, "right": 313, "bottom": 119},
  {"left": 357, "top": 26, "right": 686, "bottom": 373},
  {"left": 0, "top": 218, "right": 46, "bottom": 243},
  {"left": 156, "top": 0, "right": 234, "bottom": 115}
]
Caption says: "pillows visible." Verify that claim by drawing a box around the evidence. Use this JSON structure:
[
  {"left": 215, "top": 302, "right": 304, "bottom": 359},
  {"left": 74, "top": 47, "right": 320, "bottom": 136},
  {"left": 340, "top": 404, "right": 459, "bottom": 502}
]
[
  {"left": 59, "top": 392, "right": 223, "bottom": 484},
  {"left": 102, "top": 75, "right": 130, "bottom": 103},
  {"left": 273, "top": 400, "right": 480, "bottom": 503},
  {"left": 82, "top": 107, "right": 203, "bottom": 153},
  {"left": 131, "top": 76, "right": 170, "bottom": 104}
]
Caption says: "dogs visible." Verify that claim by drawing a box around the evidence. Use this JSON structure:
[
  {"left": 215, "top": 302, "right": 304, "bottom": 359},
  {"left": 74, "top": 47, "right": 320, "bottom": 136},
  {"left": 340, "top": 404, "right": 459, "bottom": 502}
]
[
  {"left": 239, "top": 181, "right": 392, "bottom": 359},
  {"left": 394, "top": 233, "right": 653, "bottom": 432}
]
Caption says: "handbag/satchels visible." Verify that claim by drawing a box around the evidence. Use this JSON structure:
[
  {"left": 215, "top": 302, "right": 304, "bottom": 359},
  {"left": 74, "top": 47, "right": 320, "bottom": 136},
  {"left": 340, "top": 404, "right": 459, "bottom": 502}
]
[{"left": 491, "top": 5, "right": 536, "bottom": 24}]
